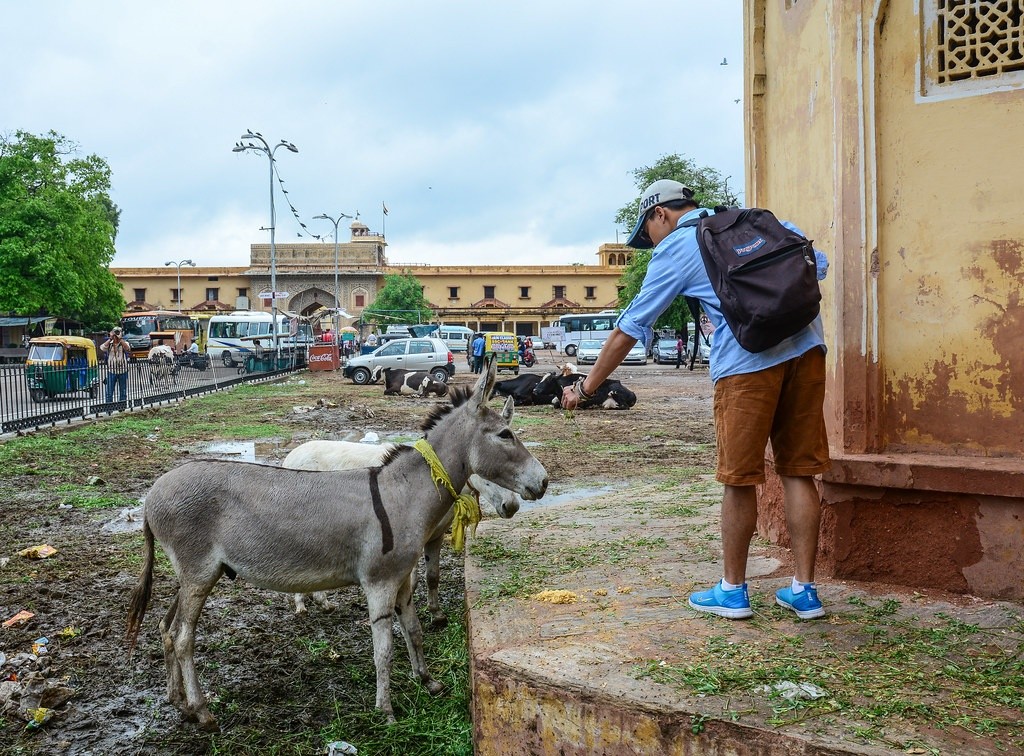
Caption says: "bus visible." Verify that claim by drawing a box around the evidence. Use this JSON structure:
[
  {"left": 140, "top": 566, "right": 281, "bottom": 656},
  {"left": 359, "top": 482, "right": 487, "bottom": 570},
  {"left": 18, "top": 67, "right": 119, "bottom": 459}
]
[
  {"left": 207, "top": 310, "right": 315, "bottom": 368},
  {"left": 385, "top": 325, "right": 476, "bottom": 351},
  {"left": 552, "top": 310, "right": 624, "bottom": 357},
  {"left": 117, "top": 310, "right": 213, "bottom": 362}
]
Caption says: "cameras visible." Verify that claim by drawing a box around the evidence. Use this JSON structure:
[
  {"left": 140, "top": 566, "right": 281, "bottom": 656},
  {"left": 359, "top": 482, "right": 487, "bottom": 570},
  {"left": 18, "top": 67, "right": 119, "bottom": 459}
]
[{"left": 114, "top": 330, "right": 120, "bottom": 335}]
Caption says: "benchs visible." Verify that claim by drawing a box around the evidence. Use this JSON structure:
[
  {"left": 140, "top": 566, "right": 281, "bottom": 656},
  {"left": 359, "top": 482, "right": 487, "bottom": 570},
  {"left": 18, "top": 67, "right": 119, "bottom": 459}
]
[{"left": 0, "top": 348, "right": 28, "bottom": 364}]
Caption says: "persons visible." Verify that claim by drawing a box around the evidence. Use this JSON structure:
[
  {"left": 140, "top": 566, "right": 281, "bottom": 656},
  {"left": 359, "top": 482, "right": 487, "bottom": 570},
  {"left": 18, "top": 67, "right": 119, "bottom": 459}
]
[
  {"left": 672, "top": 335, "right": 689, "bottom": 369},
  {"left": 472, "top": 333, "right": 485, "bottom": 374},
  {"left": 100, "top": 326, "right": 130, "bottom": 412},
  {"left": 157, "top": 339, "right": 164, "bottom": 346},
  {"left": 322, "top": 329, "right": 333, "bottom": 342},
  {"left": 517, "top": 337, "right": 525, "bottom": 355},
  {"left": 524, "top": 338, "right": 533, "bottom": 361},
  {"left": 186, "top": 338, "right": 198, "bottom": 366},
  {"left": 560, "top": 179, "right": 832, "bottom": 621},
  {"left": 337, "top": 331, "right": 377, "bottom": 359},
  {"left": 663, "top": 330, "right": 670, "bottom": 339},
  {"left": 252, "top": 341, "right": 264, "bottom": 358}
]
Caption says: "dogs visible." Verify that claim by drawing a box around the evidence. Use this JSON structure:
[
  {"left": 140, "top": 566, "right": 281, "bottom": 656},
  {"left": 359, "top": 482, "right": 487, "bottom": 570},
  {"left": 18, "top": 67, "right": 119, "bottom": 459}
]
[{"left": 557, "top": 363, "right": 577, "bottom": 376}]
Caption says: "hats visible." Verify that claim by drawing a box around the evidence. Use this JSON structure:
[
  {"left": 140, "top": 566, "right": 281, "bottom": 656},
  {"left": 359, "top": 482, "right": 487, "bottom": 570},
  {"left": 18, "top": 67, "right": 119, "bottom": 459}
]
[
  {"left": 626, "top": 179, "right": 694, "bottom": 249},
  {"left": 191, "top": 338, "right": 196, "bottom": 341}
]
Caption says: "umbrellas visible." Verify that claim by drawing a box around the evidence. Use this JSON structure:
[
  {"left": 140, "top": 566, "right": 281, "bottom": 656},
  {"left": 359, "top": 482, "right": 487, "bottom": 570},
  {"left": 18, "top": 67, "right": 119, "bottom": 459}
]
[{"left": 340, "top": 327, "right": 359, "bottom": 334}]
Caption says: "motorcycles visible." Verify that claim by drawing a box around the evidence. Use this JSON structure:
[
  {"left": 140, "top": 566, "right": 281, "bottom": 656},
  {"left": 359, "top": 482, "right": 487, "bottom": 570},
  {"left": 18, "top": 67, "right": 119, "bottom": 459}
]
[
  {"left": 518, "top": 347, "right": 535, "bottom": 367},
  {"left": 179, "top": 349, "right": 210, "bottom": 371},
  {"left": 236, "top": 332, "right": 302, "bottom": 375},
  {"left": 24, "top": 336, "right": 100, "bottom": 404},
  {"left": 468, "top": 331, "right": 520, "bottom": 375}
]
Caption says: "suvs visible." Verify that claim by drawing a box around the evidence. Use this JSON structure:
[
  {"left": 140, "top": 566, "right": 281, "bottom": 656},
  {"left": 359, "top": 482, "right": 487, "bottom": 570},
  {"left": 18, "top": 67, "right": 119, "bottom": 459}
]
[{"left": 342, "top": 338, "right": 455, "bottom": 383}]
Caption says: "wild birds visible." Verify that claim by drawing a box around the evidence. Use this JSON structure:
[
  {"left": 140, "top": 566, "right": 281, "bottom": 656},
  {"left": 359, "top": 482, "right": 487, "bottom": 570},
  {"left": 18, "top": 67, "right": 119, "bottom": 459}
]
[{"left": 720, "top": 57, "right": 742, "bottom": 105}]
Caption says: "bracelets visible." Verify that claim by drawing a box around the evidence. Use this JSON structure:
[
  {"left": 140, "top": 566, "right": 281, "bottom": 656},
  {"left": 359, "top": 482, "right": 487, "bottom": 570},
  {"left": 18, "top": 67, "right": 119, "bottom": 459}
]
[
  {"left": 571, "top": 376, "right": 598, "bottom": 402},
  {"left": 109, "top": 337, "right": 112, "bottom": 341}
]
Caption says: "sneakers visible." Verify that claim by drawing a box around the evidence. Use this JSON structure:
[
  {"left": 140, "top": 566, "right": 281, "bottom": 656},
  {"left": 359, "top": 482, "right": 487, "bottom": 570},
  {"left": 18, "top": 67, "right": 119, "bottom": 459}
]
[
  {"left": 775, "top": 585, "right": 826, "bottom": 620},
  {"left": 689, "top": 579, "right": 753, "bottom": 619}
]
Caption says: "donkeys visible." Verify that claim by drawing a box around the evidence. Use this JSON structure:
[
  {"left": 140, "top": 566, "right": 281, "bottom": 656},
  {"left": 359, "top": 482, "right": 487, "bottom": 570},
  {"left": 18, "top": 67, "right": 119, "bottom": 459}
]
[
  {"left": 124, "top": 352, "right": 549, "bottom": 735},
  {"left": 282, "top": 439, "right": 521, "bottom": 626}
]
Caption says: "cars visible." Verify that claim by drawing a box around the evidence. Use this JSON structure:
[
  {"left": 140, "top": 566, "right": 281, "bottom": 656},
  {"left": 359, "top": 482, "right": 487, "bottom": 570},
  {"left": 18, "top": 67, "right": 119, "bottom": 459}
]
[
  {"left": 517, "top": 335, "right": 545, "bottom": 350},
  {"left": 575, "top": 340, "right": 603, "bottom": 364},
  {"left": 686, "top": 334, "right": 714, "bottom": 363},
  {"left": 653, "top": 334, "right": 688, "bottom": 364},
  {"left": 621, "top": 340, "right": 647, "bottom": 365}
]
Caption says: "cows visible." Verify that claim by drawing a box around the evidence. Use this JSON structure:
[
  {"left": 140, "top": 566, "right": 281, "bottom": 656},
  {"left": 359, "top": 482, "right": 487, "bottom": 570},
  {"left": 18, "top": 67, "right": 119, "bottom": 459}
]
[
  {"left": 368, "top": 365, "right": 449, "bottom": 398},
  {"left": 488, "top": 373, "right": 636, "bottom": 410}
]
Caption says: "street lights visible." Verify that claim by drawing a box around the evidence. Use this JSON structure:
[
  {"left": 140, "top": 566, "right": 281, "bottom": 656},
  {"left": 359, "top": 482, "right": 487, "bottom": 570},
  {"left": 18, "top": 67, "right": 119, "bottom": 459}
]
[
  {"left": 164, "top": 259, "right": 197, "bottom": 313},
  {"left": 312, "top": 212, "right": 353, "bottom": 345},
  {"left": 233, "top": 133, "right": 298, "bottom": 371}
]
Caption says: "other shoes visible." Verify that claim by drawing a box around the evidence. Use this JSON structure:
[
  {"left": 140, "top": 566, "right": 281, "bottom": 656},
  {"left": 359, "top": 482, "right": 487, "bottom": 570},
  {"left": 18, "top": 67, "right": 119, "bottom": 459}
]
[
  {"left": 475, "top": 372, "right": 478, "bottom": 374},
  {"left": 186, "top": 365, "right": 189, "bottom": 368},
  {"left": 480, "top": 372, "right": 481, "bottom": 374},
  {"left": 685, "top": 363, "right": 687, "bottom": 368}
]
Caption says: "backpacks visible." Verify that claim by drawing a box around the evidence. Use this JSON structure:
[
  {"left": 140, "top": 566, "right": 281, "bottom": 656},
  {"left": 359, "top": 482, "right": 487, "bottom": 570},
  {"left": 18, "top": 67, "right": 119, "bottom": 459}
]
[{"left": 668, "top": 204, "right": 822, "bottom": 371}]
